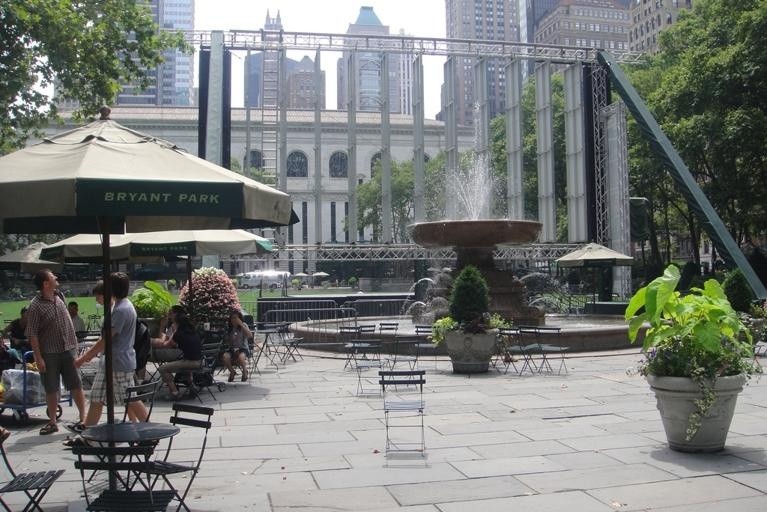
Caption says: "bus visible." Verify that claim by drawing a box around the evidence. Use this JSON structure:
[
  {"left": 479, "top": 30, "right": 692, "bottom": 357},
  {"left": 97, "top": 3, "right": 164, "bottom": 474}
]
[{"left": 239, "top": 271, "right": 292, "bottom": 288}]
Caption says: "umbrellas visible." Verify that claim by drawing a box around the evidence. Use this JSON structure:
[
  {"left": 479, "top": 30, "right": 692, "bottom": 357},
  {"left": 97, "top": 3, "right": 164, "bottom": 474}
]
[
  {"left": 554, "top": 238, "right": 635, "bottom": 304},
  {"left": 295, "top": 272, "right": 330, "bottom": 277},
  {"left": 38, "top": 229, "right": 274, "bottom": 260},
  {"left": 1, "top": 242, "right": 89, "bottom": 267},
  {"left": 0, "top": 106, "right": 301, "bottom": 490}
]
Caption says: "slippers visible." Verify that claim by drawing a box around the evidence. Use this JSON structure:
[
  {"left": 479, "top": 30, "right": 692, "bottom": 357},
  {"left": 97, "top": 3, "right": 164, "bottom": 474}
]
[{"left": 62, "top": 437, "right": 91, "bottom": 448}]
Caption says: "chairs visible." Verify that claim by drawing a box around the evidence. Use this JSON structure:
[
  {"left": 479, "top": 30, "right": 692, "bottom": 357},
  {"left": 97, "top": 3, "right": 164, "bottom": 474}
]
[
  {"left": 339, "top": 320, "right": 571, "bottom": 397},
  {"left": 129, "top": 403, "right": 216, "bottom": 511},
  {"left": 83, "top": 375, "right": 165, "bottom": 484},
  {"left": 140, "top": 322, "right": 309, "bottom": 407},
  {"left": 87, "top": 303, "right": 101, "bottom": 331},
  {"left": 1, "top": 433, "right": 66, "bottom": 512},
  {"left": 69, "top": 438, "right": 179, "bottom": 510},
  {"left": 748, "top": 345, "right": 764, "bottom": 373},
  {"left": 375, "top": 367, "right": 430, "bottom": 457}
]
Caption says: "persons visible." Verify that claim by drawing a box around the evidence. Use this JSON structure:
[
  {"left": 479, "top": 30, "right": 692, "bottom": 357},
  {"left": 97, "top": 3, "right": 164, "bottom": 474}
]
[
  {"left": 67, "top": 302, "right": 85, "bottom": 332},
  {"left": 62, "top": 272, "right": 160, "bottom": 461},
  {"left": 0, "top": 306, "right": 32, "bottom": 351},
  {"left": 0, "top": 426, "right": 11, "bottom": 445},
  {"left": 27, "top": 269, "right": 85, "bottom": 434},
  {"left": 148, "top": 305, "right": 204, "bottom": 401},
  {"left": 63, "top": 280, "right": 140, "bottom": 433},
  {"left": 223, "top": 311, "right": 253, "bottom": 381}
]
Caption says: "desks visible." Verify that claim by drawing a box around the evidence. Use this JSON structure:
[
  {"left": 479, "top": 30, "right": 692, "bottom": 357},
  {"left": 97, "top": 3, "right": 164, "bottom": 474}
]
[{"left": 79, "top": 421, "right": 179, "bottom": 493}]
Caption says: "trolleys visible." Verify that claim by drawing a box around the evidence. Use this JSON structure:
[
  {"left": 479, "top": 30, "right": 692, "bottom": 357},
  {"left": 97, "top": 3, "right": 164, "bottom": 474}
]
[
  {"left": 1, "top": 350, "right": 72, "bottom": 424},
  {"left": 175, "top": 325, "right": 225, "bottom": 392}
]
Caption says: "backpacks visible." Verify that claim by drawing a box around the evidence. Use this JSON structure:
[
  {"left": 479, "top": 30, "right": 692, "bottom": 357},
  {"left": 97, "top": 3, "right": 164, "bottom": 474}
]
[{"left": 134, "top": 316, "right": 154, "bottom": 373}]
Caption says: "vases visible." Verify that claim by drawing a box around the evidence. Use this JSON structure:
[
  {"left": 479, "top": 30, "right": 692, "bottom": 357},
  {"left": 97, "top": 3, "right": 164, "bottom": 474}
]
[{"left": 646, "top": 372, "right": 746, "bottom": 452}]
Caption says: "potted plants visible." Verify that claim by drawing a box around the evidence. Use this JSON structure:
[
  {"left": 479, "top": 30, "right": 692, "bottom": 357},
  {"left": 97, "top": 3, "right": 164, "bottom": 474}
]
[
  {"left": 423, "top": 266, "right": 517, "bottom": 374},
  {"left": 127, "top": 279, "right": 175, "bottom": 336}
]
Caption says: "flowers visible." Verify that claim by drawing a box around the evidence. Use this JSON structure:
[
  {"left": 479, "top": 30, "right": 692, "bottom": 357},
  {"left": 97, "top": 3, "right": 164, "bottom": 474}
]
[
  {"left": 621, "top": 263, "right": 767, "bottom": 452},
  {"left": 176, "top": 266, "right": 243, "bottom": 343}
]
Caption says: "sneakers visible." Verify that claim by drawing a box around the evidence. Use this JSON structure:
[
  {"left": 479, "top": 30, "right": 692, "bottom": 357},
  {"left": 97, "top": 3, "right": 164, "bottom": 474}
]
[
  {"left": 69, "top": 424, "right": 87, "bottom": 432},
  {"left": 165, "top": 392, "right": 181, "bottom": 401},
  {"left": 184, "top": 384, "right": 200, "bottom": 396}
]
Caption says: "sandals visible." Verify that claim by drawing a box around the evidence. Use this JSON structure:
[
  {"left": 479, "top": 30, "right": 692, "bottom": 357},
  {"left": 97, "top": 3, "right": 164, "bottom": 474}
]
[
  {"left": 228, "top": 371, "right": 236, "bottom": 382},
  {"left": 242, "top": 370, "right": 248, "bottom": 382},
  {"left": 40, "top": 423, "right": 58, "bottom": 435},
  {"left": 0, "top": 428, "right": 11, "bottom": 443}
]
